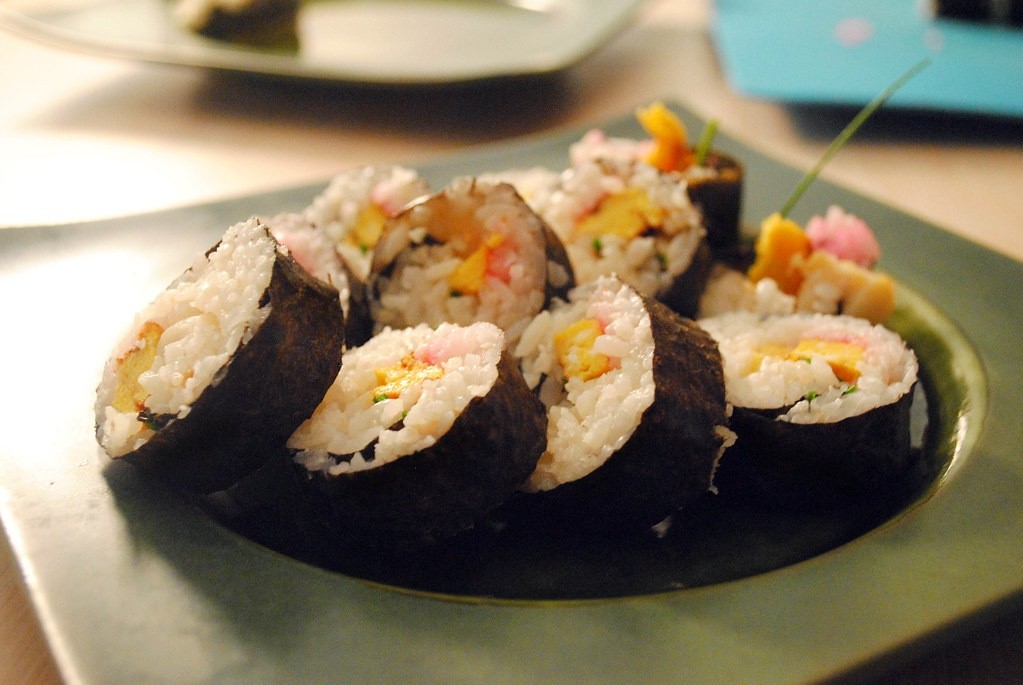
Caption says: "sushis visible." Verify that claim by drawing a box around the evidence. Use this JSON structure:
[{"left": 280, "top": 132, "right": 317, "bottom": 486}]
[{"left": 91, "top": 97, "right": 920, "bottom": 553}]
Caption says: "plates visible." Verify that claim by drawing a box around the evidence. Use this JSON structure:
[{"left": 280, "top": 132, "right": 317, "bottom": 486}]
[
  {"left": 2, "top": 98, "right": 1021, "bottom": 685},
  {"left": 710, "top": 0, "right": 1023, "bottom": 124},
  {"left": 1, "top": 0, "right": 642, "bottom": 86}
]
